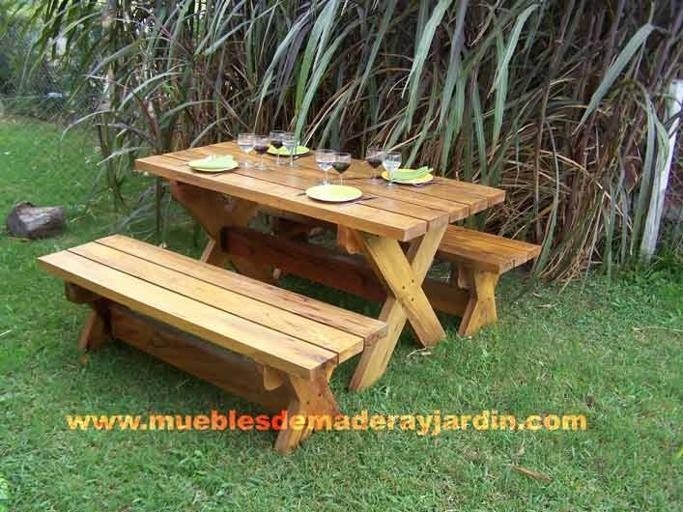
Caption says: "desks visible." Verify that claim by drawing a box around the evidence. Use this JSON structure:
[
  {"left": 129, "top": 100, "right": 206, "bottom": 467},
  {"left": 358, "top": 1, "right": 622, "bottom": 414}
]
[{"left": 134, "top": 135, "right": 505, "bottom": 395}]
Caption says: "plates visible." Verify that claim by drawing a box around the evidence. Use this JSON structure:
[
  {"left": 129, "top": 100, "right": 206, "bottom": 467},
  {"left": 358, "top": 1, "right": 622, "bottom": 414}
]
[{"left": 188, "top": 140, "right": 434, "bottom": 203}]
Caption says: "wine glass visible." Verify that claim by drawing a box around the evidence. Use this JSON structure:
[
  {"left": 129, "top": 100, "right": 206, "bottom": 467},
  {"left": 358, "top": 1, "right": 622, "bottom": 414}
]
[{"left": 238, "top": 131, "right": 402, "bottom": 188}]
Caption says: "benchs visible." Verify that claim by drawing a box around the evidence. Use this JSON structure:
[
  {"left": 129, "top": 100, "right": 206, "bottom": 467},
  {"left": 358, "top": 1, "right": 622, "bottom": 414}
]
[
  {"left": 35, "top": 232, "right": 390, "bottom": 454},
  {"left": 401, "top": 223, "right": 543, "bottom": 336}
]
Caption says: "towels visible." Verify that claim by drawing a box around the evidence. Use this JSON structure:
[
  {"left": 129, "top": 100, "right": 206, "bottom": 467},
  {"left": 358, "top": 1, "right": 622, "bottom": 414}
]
[
  {"left": 393, "top": 167, "right": 433, "bottom": 180},
  {"left": 190, "top": 153, "right": 234, "bottom": 169}
]
